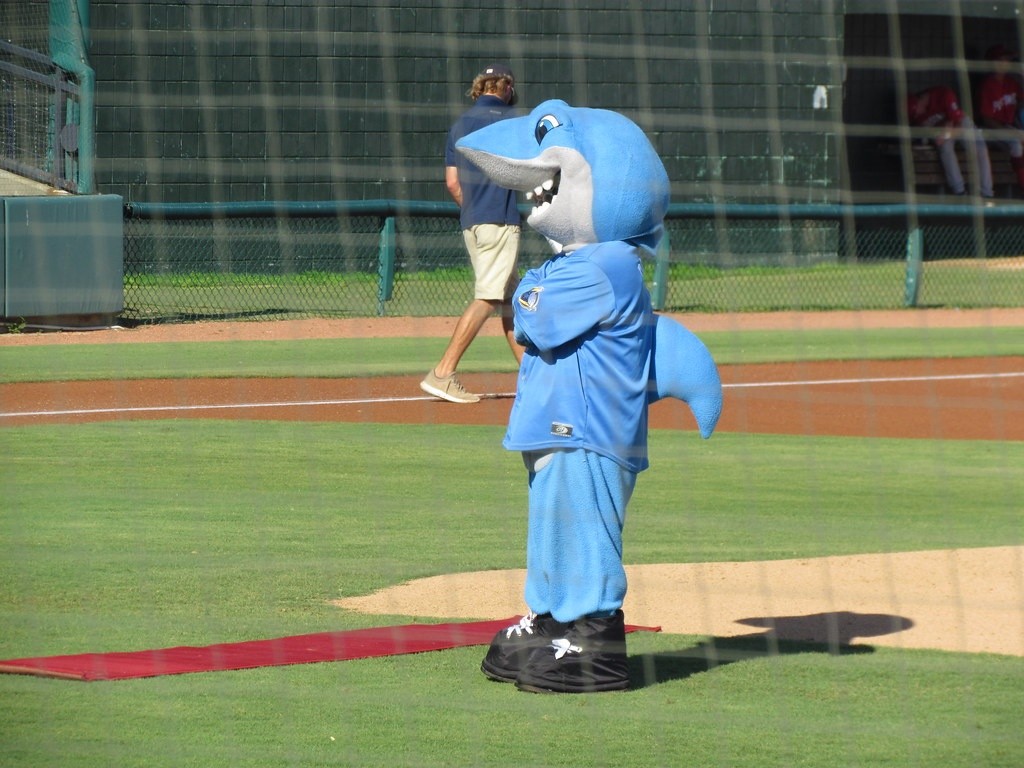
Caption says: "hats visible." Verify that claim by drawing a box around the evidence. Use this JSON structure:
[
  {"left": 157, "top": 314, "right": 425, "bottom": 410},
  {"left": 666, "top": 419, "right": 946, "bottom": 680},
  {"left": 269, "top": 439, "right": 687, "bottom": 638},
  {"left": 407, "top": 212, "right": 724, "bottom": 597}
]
[{"left": 480, "top": 63, "right": 520, "bottom": 105}]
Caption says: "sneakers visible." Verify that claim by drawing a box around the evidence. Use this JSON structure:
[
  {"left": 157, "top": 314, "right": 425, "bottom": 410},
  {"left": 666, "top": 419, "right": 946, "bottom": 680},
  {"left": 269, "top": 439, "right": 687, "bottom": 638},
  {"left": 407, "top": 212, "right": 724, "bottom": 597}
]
[{"left": 419, "top": 369, "right": 480, "bottom": 404}]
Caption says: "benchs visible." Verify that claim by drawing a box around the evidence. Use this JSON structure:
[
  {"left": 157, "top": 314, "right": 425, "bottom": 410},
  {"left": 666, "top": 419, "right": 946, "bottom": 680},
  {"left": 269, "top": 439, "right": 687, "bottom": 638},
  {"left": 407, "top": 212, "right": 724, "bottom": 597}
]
[{"left": 878, "top": 146, "right": 1017, "bottom": 200}]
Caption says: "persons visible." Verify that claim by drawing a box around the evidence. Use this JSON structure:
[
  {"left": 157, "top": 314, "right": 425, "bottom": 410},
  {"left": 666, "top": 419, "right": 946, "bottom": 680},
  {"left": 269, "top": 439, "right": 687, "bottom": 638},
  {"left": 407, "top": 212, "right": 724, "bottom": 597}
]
[
  {"left": 420, "top": 65, "right": 522, "bottom": 403},
  {"left": 903, "top": 44, "right": 1024, "bottom": 199}
]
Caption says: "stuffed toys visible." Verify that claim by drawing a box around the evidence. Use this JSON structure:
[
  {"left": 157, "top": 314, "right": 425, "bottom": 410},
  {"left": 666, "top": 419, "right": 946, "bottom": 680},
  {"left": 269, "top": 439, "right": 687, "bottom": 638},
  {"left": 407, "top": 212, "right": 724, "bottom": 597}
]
[{"left": 456, "top": 98, "right": 722, "bottom": 692}]
